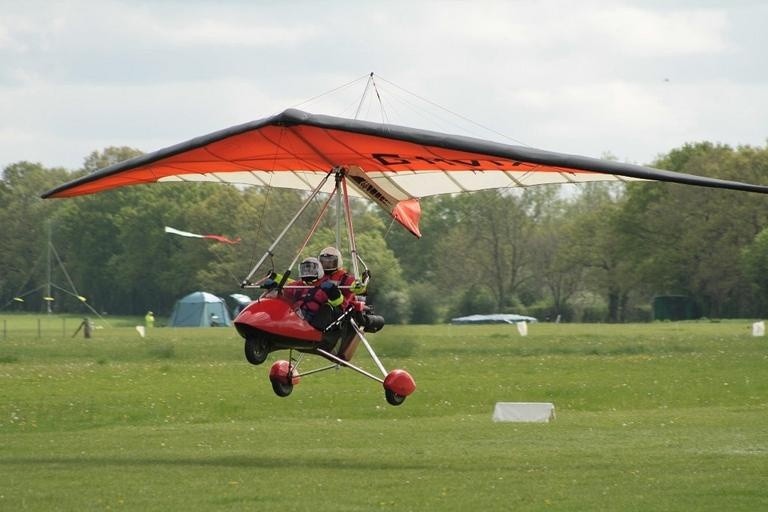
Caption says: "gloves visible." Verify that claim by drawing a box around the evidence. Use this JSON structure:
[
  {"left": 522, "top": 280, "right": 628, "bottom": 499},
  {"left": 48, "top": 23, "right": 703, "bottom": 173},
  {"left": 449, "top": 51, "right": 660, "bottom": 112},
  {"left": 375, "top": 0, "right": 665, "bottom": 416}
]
[
  {"left": 362, "top": 270, "right": 371, "bottom": 283},
  {"left": 263, "top": 279, "right": 277, "bottom": 289},
  {"left": 320, "top": 281, "right": 340, "bottom": 301},
  {"left": 266, "top": 269, "right": 277, "bottom": 278}
]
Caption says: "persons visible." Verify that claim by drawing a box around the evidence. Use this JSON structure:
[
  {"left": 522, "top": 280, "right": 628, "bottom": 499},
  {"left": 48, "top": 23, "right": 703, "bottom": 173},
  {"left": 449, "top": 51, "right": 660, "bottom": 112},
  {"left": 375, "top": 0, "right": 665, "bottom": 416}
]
[
  {"left": 145, "top": 311, "right": 156, "bottom": 338},
  {"left": 267, "top": 246, "right": 372, "bottom": 336},
  {"left": 264, "top": 257, "right": 344, "bottom": 330}
]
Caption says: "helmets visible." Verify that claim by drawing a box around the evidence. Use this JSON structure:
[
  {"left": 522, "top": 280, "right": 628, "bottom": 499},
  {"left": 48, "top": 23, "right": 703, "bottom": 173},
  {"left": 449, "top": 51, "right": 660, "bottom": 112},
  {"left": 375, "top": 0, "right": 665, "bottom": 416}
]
[
  {"left": 318, "top": 246, "right": 343, "bottom": 271},
  {"left": 298, "top": 257, "right": 325, "bottom": 280}
]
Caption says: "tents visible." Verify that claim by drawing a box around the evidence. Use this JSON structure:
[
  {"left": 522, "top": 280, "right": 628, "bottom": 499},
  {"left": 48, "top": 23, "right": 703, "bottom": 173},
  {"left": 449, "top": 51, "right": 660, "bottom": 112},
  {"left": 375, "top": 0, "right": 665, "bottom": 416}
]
[{"left": 167, "top": 290, "right": 232, "bottom": 327}]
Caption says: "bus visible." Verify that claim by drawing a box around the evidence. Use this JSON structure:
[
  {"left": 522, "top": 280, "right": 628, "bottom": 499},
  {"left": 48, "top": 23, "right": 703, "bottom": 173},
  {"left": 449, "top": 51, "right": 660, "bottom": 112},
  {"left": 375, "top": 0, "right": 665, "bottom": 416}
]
[{"left": 654, "top": 295, "right": 696, "bottom": 320}]
